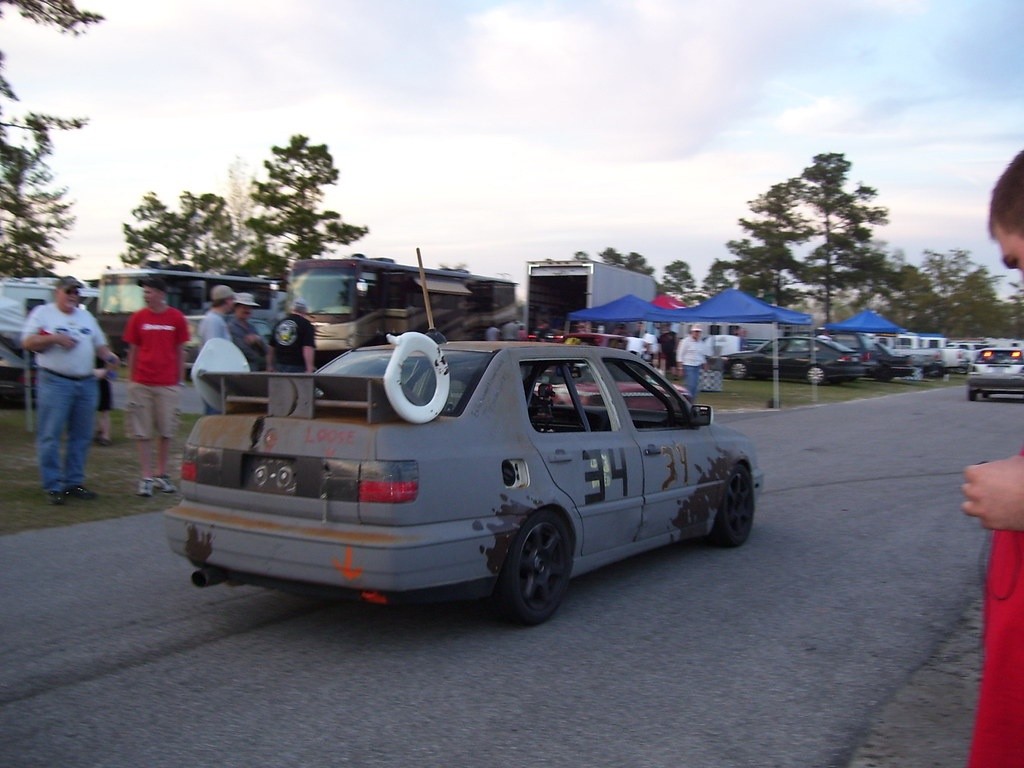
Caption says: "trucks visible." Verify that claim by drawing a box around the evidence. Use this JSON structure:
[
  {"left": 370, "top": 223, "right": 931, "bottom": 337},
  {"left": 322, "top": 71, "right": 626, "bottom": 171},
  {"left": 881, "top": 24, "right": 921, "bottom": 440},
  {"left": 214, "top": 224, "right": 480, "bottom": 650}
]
[
  {"left": 0, "top": 276, "right": 100, "bottom": 332},
  {"left": 525, "top": 260, "right": 656, "bottom": 341}
]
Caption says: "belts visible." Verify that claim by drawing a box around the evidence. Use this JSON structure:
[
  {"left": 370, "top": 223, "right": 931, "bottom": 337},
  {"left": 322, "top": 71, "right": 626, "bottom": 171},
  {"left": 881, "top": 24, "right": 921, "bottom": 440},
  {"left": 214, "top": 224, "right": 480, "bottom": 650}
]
[{"left": 43, "top": 367, "right": 95, "bottom": 380}]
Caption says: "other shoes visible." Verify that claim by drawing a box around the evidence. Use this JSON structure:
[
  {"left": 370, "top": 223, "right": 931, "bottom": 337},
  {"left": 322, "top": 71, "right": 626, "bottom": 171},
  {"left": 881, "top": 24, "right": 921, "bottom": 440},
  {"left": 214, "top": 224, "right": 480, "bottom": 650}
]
[
  {"left": 49, "top": 491, "right": 64, "bottom": 505},
  {"left": 69, "top": 487, "right": 95, "bottom": 499}
]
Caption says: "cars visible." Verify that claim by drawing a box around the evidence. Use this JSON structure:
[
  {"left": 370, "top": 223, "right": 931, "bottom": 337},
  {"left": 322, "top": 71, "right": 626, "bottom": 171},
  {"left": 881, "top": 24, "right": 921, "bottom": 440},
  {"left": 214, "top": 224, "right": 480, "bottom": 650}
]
[
  {"left": 948, "top": 339, "right": 1024, "bottom": 401},
  {"left": 163, "top": 342, "right": 764, "bottom": 625},
  {"left": 0, "top": 335, "right": 36, "bottom": 410},
  {"left": 723, "top": 334, "right": 915, "bottom": 386}
]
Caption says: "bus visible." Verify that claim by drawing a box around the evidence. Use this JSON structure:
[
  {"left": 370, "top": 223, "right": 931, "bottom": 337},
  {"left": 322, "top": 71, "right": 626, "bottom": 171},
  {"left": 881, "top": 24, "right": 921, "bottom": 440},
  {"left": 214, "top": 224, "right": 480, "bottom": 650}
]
[
  {"left": 286, "top": 255, "right": 517, "bottom": 362},
  {"left": 96, "top": 264, "right": 285, "bottom": 357}
]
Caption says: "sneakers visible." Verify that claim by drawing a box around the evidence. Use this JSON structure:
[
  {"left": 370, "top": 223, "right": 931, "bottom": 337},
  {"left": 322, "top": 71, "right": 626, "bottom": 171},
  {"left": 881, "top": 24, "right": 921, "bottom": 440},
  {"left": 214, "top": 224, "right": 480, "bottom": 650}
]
[
  {"left": 153, "top": 476, "right": 177, "bottom": 493},
  {"left": 136, "top": 480, "right": 153, "bottom": 497}
]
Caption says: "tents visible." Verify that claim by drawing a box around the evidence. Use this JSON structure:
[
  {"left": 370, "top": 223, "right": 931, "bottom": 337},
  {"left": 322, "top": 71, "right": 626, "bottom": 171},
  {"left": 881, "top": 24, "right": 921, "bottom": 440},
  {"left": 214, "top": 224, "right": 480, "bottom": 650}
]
[
  {"left": 565, "top": 295, "right": 685, "bottom": 338},
  {"left": 825, "top": 309, "right": 906, "bottom": 354},
  {"left": 642, "top": 288, "right": 816, "bottom": 408}
]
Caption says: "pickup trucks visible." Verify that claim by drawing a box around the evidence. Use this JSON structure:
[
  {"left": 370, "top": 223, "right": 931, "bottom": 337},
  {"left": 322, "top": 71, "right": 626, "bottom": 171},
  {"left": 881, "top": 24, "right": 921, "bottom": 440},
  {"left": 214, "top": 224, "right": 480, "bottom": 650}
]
[{"left": 873, "top": 336, "right": 967, "bottom": 377}]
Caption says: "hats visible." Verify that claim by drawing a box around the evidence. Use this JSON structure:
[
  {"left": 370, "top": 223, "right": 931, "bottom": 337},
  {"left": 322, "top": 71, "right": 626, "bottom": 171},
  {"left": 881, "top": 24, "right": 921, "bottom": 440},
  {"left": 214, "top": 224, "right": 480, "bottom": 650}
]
[
  {"left": 214, "top": 286, "right": 236, "bottom": 301},
  {"left": 58, "top": 277, "right": 83, "bottom": 290},
  {"left": 235, "top": 292, "right": 260, "bottom": 307},
  {"left": 137, "top": 277, "right": 165, "bottom": 291},
  {"left": 691, "top": 323, "right": 702, "bottom": 332}
]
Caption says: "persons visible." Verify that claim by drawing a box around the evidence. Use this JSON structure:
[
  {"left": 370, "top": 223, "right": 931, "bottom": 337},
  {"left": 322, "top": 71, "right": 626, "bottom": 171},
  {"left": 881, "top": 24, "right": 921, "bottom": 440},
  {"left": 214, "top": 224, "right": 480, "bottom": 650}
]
[
  {"left": 567, "top": 323, "right": 659, "bottom": 369},
  {"left": 962, "top": 152, "right": 1024, "bottom": 768},
  {"left": 485, "top": 320, "right": 527, "bottom": 341},
  {"left": 195, "top": 283, "right": 236, "bottom": 416},
  {"left": 227, "top": 293, "right": 267, "bottom": 370},
  {"left": 676, "top": 324, "right": 710, "bottom": 402},
  {"left": 736, "top": 329, "right": 749, "bottom": 350},
  {"left": 267, "top": 298, "right": 316, "bottom": 372},
  {"left": 124, "top": 277, "right": 190, "bottom": 495},
  {"left": 96, "top": 356, "right": 112, "bottom": 446},
  {"left": 658, "top": 325, "right": 678, "bottom": 378},
  {"left": 533, "top": 319, "right": 550, "bottom": 341},
  {"left": 22, "top": 276, "right": 119, "bottom": 504}
]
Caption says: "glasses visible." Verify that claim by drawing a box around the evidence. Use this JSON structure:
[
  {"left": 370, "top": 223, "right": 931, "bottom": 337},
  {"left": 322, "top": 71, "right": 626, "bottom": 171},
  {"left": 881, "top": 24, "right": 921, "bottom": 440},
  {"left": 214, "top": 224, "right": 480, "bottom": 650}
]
[{"left": 62, "top": 289, "right": 80, "bottom": 295}]
